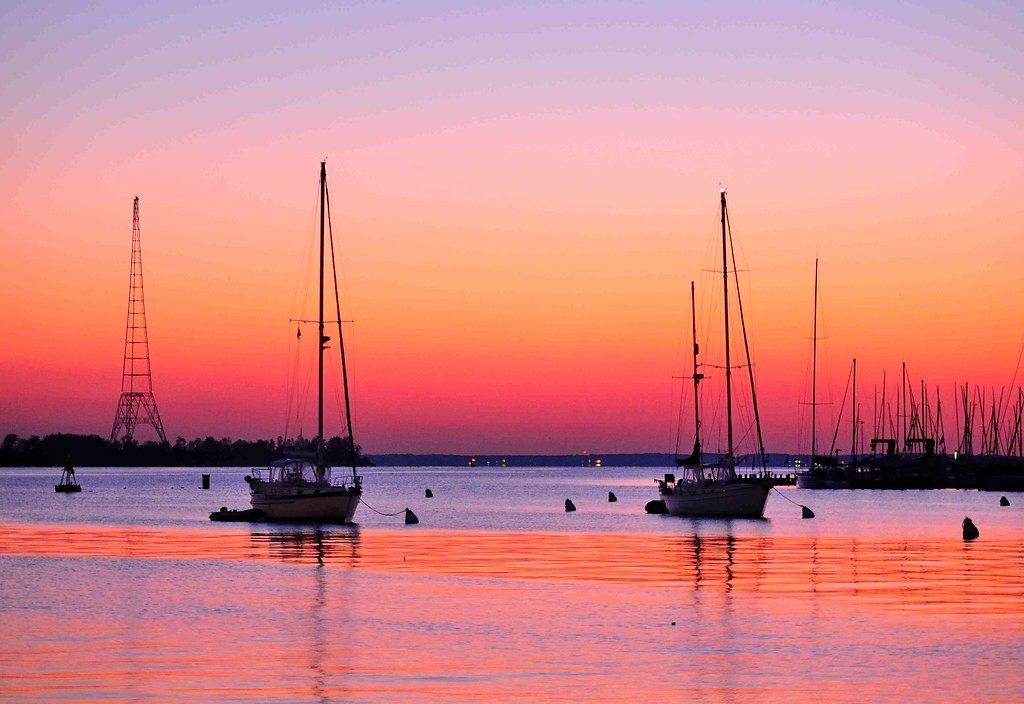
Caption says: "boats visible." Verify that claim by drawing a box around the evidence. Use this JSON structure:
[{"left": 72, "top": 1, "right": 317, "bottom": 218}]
[{"left": 54, "top": 454, "right": 82, "bottom": 493}]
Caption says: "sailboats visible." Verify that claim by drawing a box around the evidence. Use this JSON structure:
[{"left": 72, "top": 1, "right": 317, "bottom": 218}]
[
  {"left": 792, "top": 257, "right": 1023, "bottom": 489},
  {"left": 245, "top": 159, "right": 365, "bottom": 524},
  {"left": 656, "top": 188, "right": 800, "bottom": 523}
]
